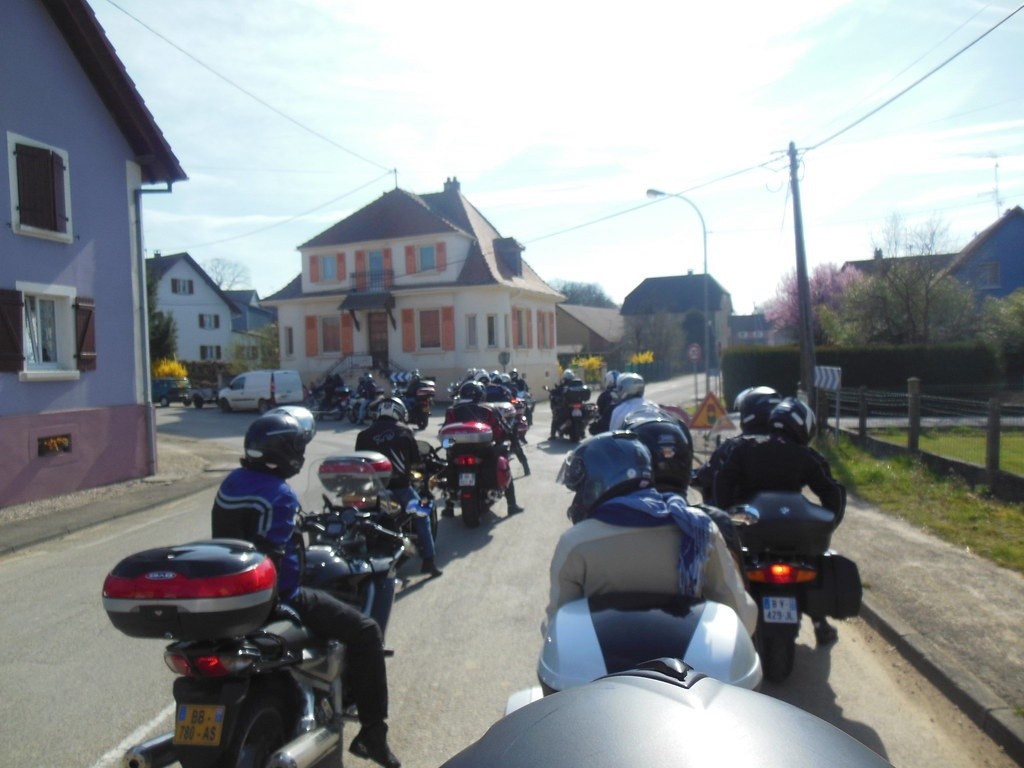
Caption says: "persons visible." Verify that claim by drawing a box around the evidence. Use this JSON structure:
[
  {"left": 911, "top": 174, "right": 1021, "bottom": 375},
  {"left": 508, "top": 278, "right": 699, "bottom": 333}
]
[
  {"left": 539, "top": 408, "right": 758, "bottom": 641},
  {"left": 466, "top": 367, "right": 532, "bottom": 476},
  {"left": 211, "top": 405, "right": 400, "bottom": 768},
  {"left": 357, "top": 373, "right": 376, "bottom": 424},
  {"left": 316, "top": 372, "right": 344, "bottom": 420},
  {"left": 355, "top": 396, "right": 443, "bottom": 575},
  {"left": 609, "top": 373, "right": 661, "bottom": 430},
  {"left": 442, "top": 380, "right": 524, "bottom": 517},
  {"left": 547, "top": 370, "right": 586, "bottom": 440},
  {"left": 403, "top": 368, "right": 420, "bottom": 411},
  {"left": 597, "top": 371, "right": 620, "bottom": 416},
  {"left": 696, "top": 385, "right": 846, "bottom": 642}
]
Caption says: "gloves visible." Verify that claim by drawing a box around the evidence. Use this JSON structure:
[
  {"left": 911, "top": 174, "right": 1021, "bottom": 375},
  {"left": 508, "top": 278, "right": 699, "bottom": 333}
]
[{"left": 268, "top": 598, "right": 303, "bottom": 626}]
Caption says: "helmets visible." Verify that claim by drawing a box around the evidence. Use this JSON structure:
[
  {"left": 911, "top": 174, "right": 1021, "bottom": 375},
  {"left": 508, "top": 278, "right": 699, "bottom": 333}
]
[
  {"left": 459, "top": 381, "right": 487, "bottom": 404},
  {"left": 244, "top": 414, "right": 307, "bottom": 477},
  {"left": 768, "top": 396, "right": 819, "bottom": 445},
  {"left": 466, "top": 367, "right": 518, "bottom": 384},
  {"left": 615, "top": 372, "right": 645, "bottom": 401},
  {"left": 627, "top": 419, "right": 693, "bottom": 495},
  {"left": 740, "top": 385, "right": 781, "bottom": 435},
  {"left": 360, "top": 377, "right": 364, "bottom": 382},
  {"left": 566, "top": 431, "right": 654, "bottom": 526},
  {"left": 335, "top": 374, "right": 340, "bottom": 379},
  {"left": 412, "top": 370, "right": 418, "bottom": 375},
  {"left": 564, "top": 368, "right": 574, "bottom": 381},
  {"left": 412, "top": 375, "right": 420, "bottom": 384},
  {"left": 369, "top": 394, "right": 408, "bottom": 422},
  {"left": 363, "top": 372, "right": 372, "bottom": 380},
  {"left": 326, "top": 375, "right": 332, "bottom": 381},
  {"left": 606, "top": 371, "right": 621, "bottom": 388}
]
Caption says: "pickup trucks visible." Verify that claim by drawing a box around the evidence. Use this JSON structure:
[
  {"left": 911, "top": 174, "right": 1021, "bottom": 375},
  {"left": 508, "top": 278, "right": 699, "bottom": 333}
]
[{"left": 152, "top": 377, "right": 213, "bottom": 410}]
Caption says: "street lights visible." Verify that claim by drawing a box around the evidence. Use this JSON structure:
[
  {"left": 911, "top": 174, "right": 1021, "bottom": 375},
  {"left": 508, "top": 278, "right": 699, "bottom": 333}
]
[{"left": 647, "top": 189, "right": 710, "bottom": 395}]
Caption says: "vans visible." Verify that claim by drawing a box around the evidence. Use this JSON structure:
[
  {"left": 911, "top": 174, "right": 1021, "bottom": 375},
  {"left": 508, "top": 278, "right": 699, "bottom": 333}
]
[{"left": 218, "top": 370, "right": 304, "bottom": 414}]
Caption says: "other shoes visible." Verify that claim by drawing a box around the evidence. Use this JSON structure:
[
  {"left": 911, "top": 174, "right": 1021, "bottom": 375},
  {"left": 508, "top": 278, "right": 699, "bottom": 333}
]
[
  {"left": 815, "top": 626, "right": 837, "bottom": 647},
  {"left": 421, "top": 560, "right": 443, "bottom": 576},
  {"left": 523, "top": 463, "right": 531, "bottom": 474}
]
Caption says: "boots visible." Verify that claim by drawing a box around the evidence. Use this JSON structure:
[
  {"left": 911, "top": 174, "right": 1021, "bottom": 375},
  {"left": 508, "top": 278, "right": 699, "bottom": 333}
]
[
  {"left": 441, "top": 499, "right": 455, "bottom": 516},
  {"left": 505, "top": 480, "right": 524, "bottom": 515},
  {"left": 371, "top": 605, "right": 394, "bottom": 656}
]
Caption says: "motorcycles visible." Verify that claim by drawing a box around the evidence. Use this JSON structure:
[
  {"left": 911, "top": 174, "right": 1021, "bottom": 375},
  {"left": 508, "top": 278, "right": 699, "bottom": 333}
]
[
  {"left": 437, "top": 373, "right": 531, "bottom": 528},
  {"left": 319, "top": 436, "right": 456, "bottom": 567},
  {"left": 689, "top": 412, "right": 837, "bottom": 682},
  {"left": 348, "top": 379, "right": 437, "bottom": 430},
  {"left": 536, "top": 487, "right": 764, "bottom": 693},
  {"left": 543, "top": 384, "right": 600, "bottom": 443},
  {"left": 103, "top": 456, "right": 416, "bottom": 768},
  {"left": 306, "top": 384, "right": 352, "bottom": 421}
]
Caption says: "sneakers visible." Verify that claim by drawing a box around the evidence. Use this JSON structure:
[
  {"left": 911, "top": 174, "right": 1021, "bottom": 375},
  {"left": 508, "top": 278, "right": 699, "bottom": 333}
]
[{"left": 349, "top": 724, "right": 400, "bottom": 768}]
